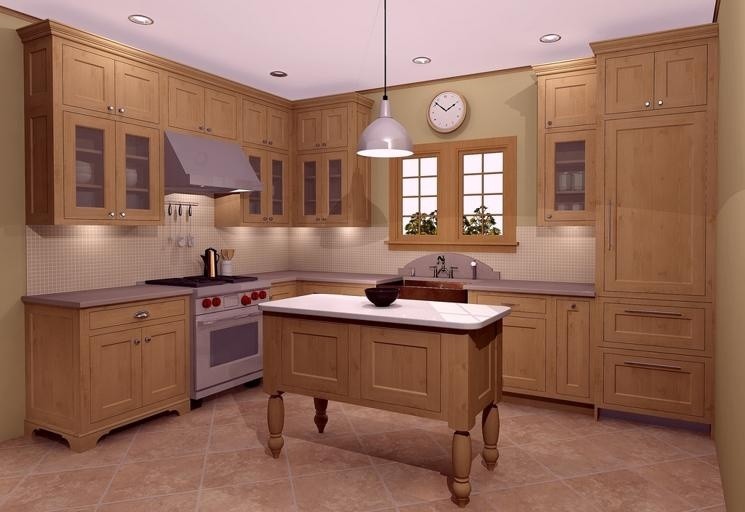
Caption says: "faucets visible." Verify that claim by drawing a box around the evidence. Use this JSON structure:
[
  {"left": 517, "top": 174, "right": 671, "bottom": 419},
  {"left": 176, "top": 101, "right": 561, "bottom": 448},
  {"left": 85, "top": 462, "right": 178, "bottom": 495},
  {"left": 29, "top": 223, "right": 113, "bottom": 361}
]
[{"left": 436, "top": 254, "right": 446, "bottom": 272}]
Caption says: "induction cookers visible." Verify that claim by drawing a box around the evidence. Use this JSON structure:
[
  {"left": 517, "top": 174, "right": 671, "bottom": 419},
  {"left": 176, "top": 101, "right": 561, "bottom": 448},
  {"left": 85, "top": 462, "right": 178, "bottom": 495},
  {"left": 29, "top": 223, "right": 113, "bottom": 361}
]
[{"left": 135, "top": 274, "right": 271, "bottom": 315}]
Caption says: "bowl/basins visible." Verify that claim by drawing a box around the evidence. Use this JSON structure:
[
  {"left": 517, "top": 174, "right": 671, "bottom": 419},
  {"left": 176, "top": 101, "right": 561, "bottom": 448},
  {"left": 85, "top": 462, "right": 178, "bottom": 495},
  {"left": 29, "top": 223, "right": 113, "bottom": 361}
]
[
  {"left": 126, "top": 169, "right": 137, "bottom": 188},
  {"left": 365, "top": 287, "right": 400, "bottom": 307},
  {"left": 76, "top": 161, "right": 92, "bottom": 184}
]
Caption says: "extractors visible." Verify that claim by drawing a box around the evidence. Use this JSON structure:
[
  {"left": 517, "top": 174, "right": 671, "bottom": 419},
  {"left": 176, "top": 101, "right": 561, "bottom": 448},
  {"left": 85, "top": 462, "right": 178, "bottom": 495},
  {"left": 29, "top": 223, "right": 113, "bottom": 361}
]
[{"left": 163, "top": 130, "right": 264, "bottom": 199}]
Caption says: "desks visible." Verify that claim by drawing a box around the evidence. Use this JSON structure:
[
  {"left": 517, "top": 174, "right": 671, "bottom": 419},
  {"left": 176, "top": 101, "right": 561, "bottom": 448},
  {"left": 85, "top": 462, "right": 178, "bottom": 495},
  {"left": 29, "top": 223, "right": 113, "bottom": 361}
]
[{"left": 256, "top": 291, "right": 513, "bottom": 507}]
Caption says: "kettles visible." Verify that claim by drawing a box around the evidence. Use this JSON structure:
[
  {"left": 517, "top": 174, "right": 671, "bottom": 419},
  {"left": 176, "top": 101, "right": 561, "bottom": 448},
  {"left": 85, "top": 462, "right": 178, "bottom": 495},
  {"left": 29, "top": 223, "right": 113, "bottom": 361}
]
[{"left": 200, "top": 246, "right": 219, "bottom": 280}]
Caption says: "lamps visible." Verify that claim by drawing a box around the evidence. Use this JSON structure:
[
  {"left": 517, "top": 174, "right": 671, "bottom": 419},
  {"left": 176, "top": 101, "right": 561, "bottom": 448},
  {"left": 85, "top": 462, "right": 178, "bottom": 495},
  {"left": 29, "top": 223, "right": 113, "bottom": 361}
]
[{"left": 355, "top": 1, "right": 416, "bottom": 160}]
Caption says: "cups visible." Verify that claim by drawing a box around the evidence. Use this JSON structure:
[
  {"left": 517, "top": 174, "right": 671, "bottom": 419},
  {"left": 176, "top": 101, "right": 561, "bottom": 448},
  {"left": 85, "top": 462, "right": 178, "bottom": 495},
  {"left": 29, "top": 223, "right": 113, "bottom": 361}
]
[
  {"left": 554, "top": 169, "right": 586, "bottom": 211},
  {"left": 222, "top": 260, "right": 233, "bottom": 276}
]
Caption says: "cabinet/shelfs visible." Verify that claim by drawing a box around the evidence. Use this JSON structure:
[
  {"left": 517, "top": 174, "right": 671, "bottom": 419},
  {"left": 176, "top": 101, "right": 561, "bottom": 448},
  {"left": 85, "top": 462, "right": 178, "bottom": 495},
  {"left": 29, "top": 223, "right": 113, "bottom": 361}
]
[
  {"left": 296, "top": 279, "right": 376, "bottom": 298},
  {"left": 215, "top": 79, "right": 291, "bottom": 227},
  {"left": 268, "top": 282, "right": 296, "bottom": 304},
  {"left": 24, "top": 290, "right": 191, "bottom": 452},
  {"left": 288, "top": 94, "right": 374, "bottom": 227},
  {"left": 532, "top": 60, "right": 597, "bottom": 226},
  {"left": 13, "top": 19, "right": 165, "bottom": 227},
  {"left": 163, "top": 58, "right": 242, "bottom": 143},
  {"left": 594, "top": 26, "right": 715, "bottom": 432},
  {"left": 467, "top": 288, "right": 595, "bottom": 405}
]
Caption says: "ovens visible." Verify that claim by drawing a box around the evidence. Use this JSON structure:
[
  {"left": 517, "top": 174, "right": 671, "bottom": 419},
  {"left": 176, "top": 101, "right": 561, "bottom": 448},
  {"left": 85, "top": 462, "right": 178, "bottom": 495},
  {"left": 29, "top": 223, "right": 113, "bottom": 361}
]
[{"left": 190, "top": 305, "right": 263, "bottom": 399}]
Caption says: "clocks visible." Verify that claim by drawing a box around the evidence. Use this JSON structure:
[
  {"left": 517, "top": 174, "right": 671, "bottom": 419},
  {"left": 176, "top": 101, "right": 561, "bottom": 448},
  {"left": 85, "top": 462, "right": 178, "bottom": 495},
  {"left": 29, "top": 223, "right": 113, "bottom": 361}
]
[{"left": 425, "top": 89, "right": 468, "bottom": 133}]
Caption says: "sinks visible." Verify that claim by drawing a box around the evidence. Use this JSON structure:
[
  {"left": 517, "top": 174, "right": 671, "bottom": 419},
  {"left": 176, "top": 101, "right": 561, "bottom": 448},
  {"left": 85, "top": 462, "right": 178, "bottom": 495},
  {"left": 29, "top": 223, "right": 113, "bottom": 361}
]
[{"left": 376, "top": 275, "right": 471, "bottom": 304}]
